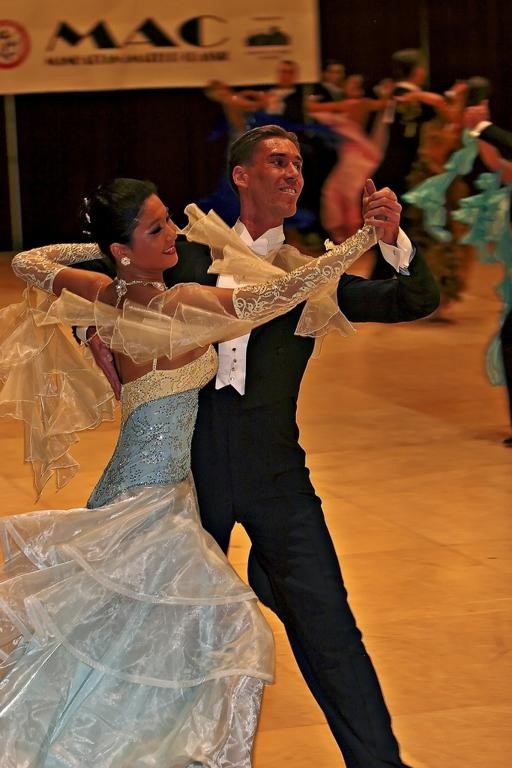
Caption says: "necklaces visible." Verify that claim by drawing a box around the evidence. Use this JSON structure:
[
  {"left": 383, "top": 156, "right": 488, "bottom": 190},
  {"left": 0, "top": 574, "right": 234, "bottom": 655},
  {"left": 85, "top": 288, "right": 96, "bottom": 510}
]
[{"left": 111, "top": 275, "right": 167, "bottom": 307}]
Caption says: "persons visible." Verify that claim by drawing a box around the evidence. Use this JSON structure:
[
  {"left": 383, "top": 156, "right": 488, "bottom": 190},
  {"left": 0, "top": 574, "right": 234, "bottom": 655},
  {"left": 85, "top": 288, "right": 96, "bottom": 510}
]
[
  {"left": 204, "top": 49, "right": 512, "bottom": 324},
  {"left": 456, "top": 99, "right": 510, "bottom": 444},
  {"left": 72, "top": 125, "right": 439, "bottom": 768},
  {"left": 0, "top": 176, "right": 383, "bottom": 768}
]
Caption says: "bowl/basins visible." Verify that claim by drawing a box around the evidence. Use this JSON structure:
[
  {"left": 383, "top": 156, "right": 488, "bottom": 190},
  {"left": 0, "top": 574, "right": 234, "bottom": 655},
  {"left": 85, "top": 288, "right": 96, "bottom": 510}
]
[{"left": 111, "top": 275, "right": 167, "bottom": 307}]
